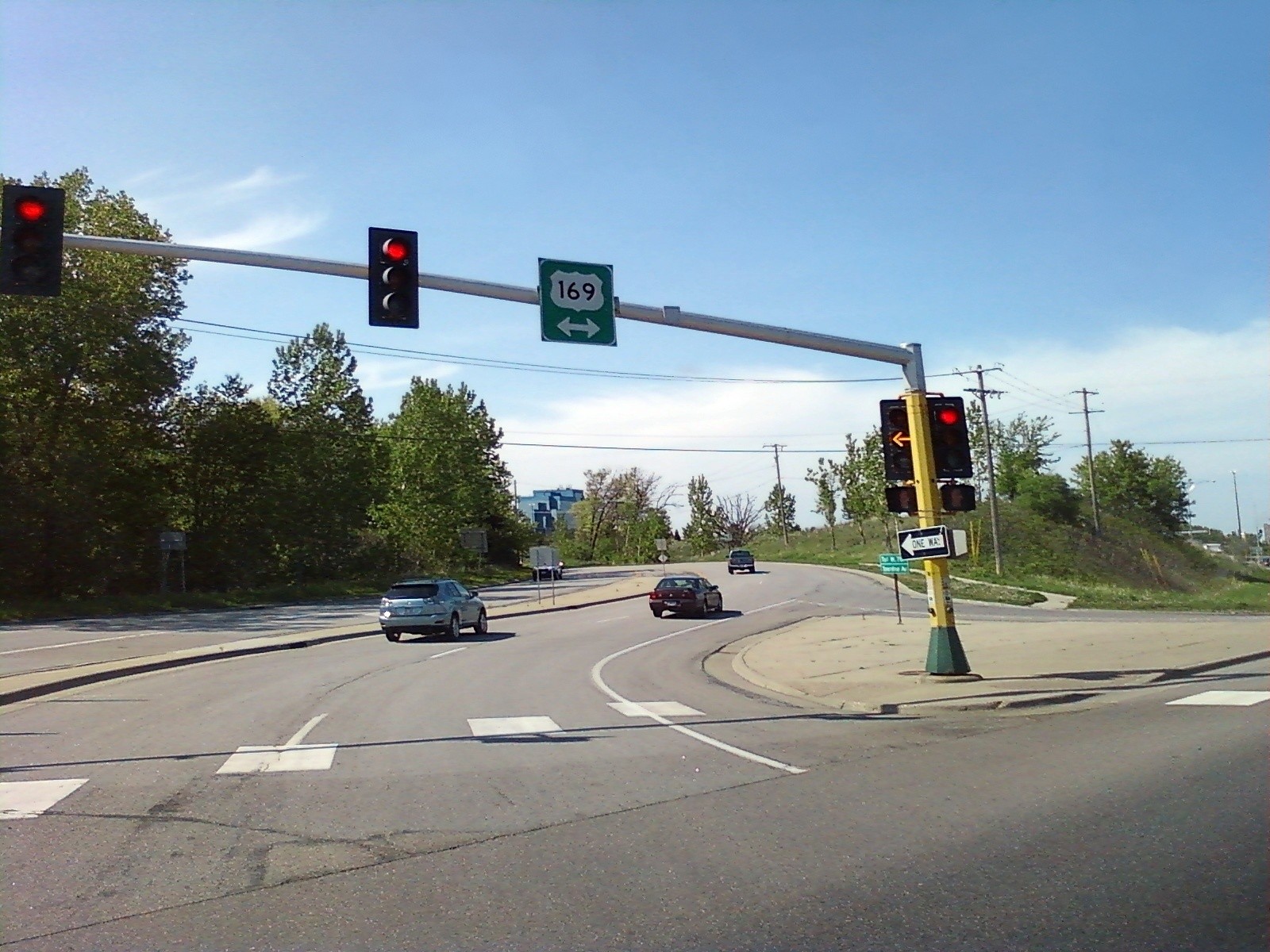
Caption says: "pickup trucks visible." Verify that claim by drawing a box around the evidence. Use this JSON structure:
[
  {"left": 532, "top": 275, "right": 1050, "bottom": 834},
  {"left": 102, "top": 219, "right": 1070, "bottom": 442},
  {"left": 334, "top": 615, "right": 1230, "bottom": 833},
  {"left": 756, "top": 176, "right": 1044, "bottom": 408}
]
[{"left": 725, "top": 549, "right": 755, "bottom": 574}]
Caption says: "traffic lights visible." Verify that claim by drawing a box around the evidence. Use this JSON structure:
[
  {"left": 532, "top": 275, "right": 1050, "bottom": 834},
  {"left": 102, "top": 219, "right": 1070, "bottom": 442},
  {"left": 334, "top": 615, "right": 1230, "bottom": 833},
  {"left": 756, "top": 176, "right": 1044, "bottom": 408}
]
[
  {"left": 940, "top": 484, "right": 976, "bottom": 512},
  {"left": 924, "top": 396, "right": 973, "bottom": 478},
  {"left": 0, "top": 184, "right": 65, "bottom": 297},
  {"left": 367, "top": 226, "right": 419, "bottom": 328},
  {"left": 880, "top": 400, "right": 914, "bottom": 480},
  {"left": 885, "top": 487, "right": 917, "bottom": 513}
]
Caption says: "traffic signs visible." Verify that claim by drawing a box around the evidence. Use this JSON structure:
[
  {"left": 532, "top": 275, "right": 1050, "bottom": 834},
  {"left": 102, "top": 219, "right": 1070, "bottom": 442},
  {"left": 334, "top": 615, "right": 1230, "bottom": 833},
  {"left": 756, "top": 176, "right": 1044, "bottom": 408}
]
[{"left": 897, "top": 524, "right": 951, "bottom": 561}]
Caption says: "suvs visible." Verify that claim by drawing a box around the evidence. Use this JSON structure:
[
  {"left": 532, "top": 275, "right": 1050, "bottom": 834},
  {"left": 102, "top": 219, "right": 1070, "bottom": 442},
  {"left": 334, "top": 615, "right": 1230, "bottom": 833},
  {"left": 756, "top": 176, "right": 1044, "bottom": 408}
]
[{"left": 378, "top": 578, "right": 488, "bottom": 642}]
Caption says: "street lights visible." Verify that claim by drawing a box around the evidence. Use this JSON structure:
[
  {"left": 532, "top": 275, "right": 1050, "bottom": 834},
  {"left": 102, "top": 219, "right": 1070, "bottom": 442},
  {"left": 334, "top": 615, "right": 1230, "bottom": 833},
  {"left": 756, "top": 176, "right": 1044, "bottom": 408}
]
[
  {"left": 1232, "top": 470, "right": 1245, "bottom": 540},
  {"left": 762, "top": 444, "right": 788, "bottom": 547}
]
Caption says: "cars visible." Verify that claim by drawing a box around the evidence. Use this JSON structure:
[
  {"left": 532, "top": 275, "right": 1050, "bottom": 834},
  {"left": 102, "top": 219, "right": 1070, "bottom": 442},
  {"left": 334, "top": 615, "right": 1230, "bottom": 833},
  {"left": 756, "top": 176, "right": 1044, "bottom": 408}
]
[
  {"left": 649, "top": 576, "right": 722, "bottom": 618},
  {"left": 532, "top": 559, "right": 564, "bottom": 581}
]
